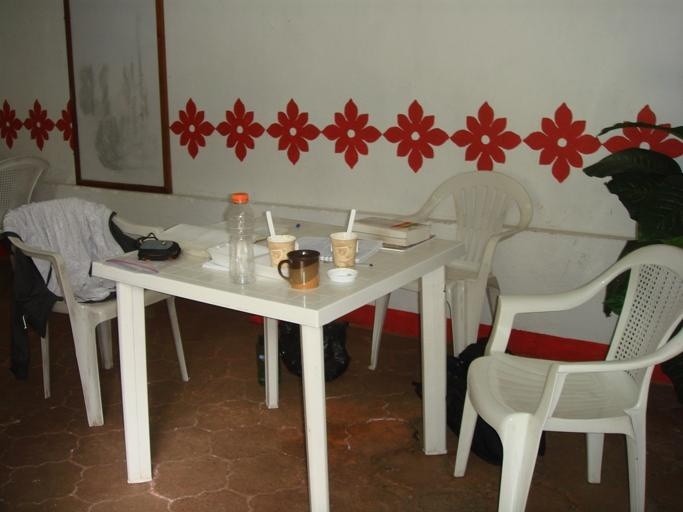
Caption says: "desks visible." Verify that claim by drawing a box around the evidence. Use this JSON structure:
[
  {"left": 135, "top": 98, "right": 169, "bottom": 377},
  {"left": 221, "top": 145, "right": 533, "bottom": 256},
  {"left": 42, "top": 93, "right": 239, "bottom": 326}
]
[{"left": 89, "top": 214, "right": 469, "bottom": 510}]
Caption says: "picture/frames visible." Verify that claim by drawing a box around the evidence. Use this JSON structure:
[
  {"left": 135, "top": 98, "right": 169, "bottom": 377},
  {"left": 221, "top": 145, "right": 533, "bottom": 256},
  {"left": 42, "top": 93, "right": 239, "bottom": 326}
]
[{"left": 61, "top": 0, "right": 174, "bottom": 195}]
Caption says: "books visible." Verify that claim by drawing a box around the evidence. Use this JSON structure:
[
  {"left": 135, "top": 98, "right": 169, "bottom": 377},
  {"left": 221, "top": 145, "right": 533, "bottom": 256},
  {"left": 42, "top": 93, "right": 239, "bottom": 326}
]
[
  {"left": 296, "top": 235, "right": 383, "bottom": 263},
  {"left": 158, "top": 220, "right": 266, "bottom": 259},
  {"left": 350, "top": 231, "right": 435, "bottom": 252},
  {"left": 349, "top": 217, "right": 431, "bottom": 238}
]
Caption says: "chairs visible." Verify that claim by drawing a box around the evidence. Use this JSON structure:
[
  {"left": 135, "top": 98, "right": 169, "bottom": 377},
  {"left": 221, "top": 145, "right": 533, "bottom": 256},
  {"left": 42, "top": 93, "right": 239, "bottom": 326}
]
[
  {"left": 0, "top": 154, "right": 50, "bottom": 234},
  {"left": 1, "top": 197, "right": 191, "bottom": 430},
  {"left": 449, "top": 241, "right": 682, "bottom": 512},
  {"left": 364, "top": 168, "right": 535, "bottom": 373}
]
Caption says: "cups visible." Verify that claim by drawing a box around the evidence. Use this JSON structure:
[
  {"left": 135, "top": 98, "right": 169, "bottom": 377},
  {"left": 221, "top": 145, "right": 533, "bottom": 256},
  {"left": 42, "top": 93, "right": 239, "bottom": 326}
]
[
  {"left": 278, "top": 250, "right": 321, "bottom": 289},
  {"left": 328, "top": 232, "right": 358, "bottom": 267},
  {"left": 267, "top": 235, "right": 301, "bottom": 266}
]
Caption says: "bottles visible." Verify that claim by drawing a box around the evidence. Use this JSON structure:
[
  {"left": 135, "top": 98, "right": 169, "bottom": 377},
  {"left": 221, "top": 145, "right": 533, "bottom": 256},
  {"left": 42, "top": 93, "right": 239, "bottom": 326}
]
[{"left": 227, "top": 193, "right": 256, "bottom": 285}]
